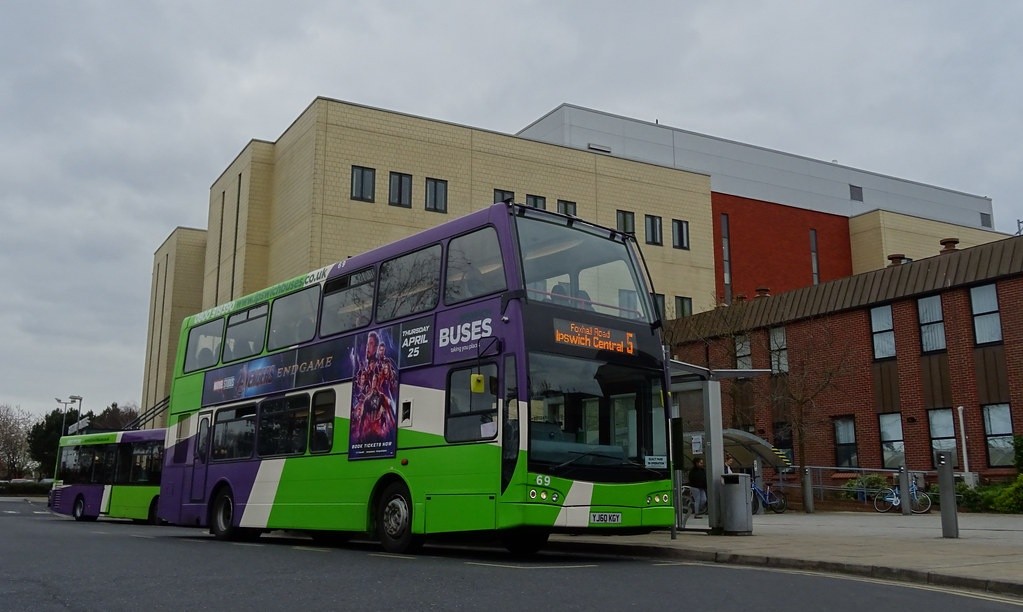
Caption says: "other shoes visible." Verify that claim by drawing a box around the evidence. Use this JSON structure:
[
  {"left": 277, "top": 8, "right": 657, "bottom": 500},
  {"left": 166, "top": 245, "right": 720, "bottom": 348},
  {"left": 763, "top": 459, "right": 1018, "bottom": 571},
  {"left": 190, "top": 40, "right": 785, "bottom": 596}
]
[{"left": 695, "top": 515, "right": 703, "bottom": 519}]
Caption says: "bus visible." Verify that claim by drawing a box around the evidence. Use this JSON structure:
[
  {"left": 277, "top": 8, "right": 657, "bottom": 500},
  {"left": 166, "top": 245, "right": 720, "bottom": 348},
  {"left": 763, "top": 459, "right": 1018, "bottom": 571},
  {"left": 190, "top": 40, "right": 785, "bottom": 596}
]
[
  {"left": 47, "top": 428, "right": 171, "bottom": 526},
  {"left": 156, "top": 198, "right": 680, "bottom": 553}
]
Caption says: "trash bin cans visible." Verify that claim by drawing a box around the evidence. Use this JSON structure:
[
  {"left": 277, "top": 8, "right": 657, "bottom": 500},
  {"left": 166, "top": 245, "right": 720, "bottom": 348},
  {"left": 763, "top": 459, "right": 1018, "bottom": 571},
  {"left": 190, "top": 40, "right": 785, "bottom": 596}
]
[{"left": 720, "top": 473, "right": 753, "bottom": 536}]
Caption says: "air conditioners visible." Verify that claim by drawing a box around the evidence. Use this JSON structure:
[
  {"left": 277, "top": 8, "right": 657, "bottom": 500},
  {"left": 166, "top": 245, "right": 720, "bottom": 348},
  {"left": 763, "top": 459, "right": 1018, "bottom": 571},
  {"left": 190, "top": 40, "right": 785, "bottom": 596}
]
[
  {"left": 953, "top": 471, "right": 979, "bottom": 488},
  {"left": 893, "top": 472, "right": 925, "bottom": 488}
]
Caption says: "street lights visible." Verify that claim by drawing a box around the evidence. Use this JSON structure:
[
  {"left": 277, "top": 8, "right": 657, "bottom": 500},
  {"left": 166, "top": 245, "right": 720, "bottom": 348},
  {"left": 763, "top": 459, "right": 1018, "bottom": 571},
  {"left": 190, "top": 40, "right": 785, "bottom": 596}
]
[
  {"left": 68, "top": 395, "right": 83, "bottom": 435},
  {"left": 53, "top": 397, "right": 77, "bottom": 482}
]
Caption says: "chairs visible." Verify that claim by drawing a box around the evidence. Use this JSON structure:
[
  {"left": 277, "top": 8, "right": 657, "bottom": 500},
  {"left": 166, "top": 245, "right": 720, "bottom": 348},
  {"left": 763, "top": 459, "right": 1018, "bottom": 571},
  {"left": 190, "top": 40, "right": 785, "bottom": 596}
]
[
  {"left": 226, "top": 430, "right": 328, "bottom": 462},
  {"left": 356, "top": 278, "right": 596, "bottom": 329},
  {"left": 196, "top": 315, "right": 343, "bottom": 369}
]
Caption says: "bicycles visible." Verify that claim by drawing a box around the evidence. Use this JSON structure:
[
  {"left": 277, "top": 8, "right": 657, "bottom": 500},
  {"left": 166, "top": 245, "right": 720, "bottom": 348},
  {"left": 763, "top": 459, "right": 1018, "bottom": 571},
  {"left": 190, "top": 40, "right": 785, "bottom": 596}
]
[
  {"left": 872, "top": 470, "right": 933, "bottom": 514},
  {"left": 679, "top": 484, "right": 708, "bottom": 516},
  {"left": 750, "top": 476, "right": 787, "bottom": 515}
]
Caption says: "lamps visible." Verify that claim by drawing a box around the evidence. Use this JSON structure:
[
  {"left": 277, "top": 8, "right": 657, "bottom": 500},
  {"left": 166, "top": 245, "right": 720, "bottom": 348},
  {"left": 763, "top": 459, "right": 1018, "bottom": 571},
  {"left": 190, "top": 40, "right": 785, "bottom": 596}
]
[
  {"left": 906, "top": 416, "right": 917, "bottom": 422},
  {"left": 758, "top": 428, "right": 766, "bottom": 434}
]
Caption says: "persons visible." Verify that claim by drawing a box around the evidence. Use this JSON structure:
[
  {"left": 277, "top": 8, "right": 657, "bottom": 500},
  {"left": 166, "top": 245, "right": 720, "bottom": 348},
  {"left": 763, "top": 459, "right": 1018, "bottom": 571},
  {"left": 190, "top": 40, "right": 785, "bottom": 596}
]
[
  {"left": 724, "top": 452, "right": 734, "bottom": 474},
  {"left": 351, "top": 331, "right": 398, "bottom": 443},
  {"left": 689, "top": 457, "right": 707, "bottom": 519}
]
